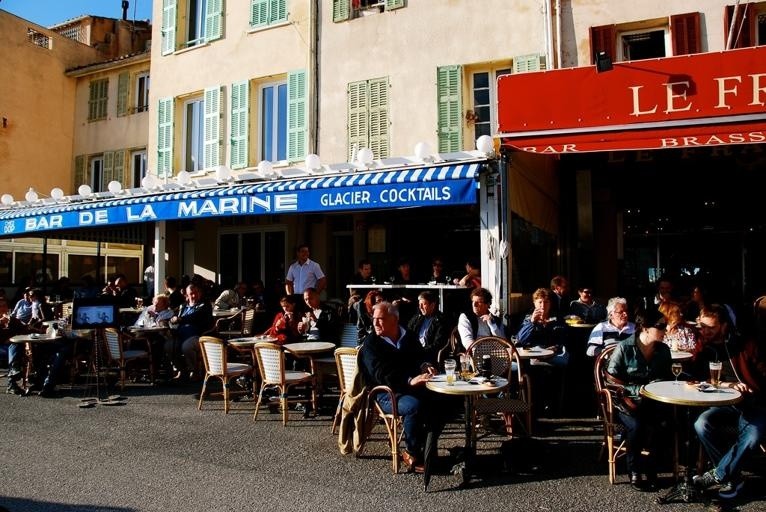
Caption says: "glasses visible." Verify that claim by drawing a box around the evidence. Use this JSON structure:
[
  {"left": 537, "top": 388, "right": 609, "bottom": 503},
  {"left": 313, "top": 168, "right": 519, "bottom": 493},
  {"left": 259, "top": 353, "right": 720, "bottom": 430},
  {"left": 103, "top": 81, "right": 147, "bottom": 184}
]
[{"left": 652, "top": 322, "right": 667, "bottom": 331}]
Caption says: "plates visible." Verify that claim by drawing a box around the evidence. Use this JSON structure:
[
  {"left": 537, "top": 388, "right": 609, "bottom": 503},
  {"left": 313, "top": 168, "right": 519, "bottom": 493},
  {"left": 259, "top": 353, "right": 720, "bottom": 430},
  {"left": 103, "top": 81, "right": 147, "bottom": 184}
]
[{"left": 527, "top": 349, "right": 545, "bottom": 352}]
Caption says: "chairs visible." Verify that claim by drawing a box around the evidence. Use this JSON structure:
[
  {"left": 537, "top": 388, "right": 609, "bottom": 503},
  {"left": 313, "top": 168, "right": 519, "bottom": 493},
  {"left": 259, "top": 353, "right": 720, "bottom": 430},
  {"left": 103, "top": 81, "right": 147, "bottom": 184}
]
[
  {"left": 0, "top": 297, "right": 335, "bottom": 425},
  {"left": 336, "top": 281, "right": 764, "bottom": 492}
]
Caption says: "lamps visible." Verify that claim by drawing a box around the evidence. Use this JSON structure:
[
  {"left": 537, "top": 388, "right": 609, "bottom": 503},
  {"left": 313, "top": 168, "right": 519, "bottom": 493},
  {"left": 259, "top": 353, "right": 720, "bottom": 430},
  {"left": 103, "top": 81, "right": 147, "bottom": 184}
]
[
  {"left": 257, "top": 135, "right": 499, "bottom": 183},
  {"left": 0, "top": 164, "right": 231, "bottom": 207},
  {"left": 594, "top": 51, "right": 615, "bottom": 75}
]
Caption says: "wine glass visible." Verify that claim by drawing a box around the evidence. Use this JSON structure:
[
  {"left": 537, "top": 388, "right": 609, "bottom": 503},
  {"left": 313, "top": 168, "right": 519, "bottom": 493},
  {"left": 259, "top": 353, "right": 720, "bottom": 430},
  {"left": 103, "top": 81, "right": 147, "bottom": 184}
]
[
  {"left": 372, "top": 275, "right": 452, "bottom": 286},
  {"left": 138, "top": 298, "right": 144, "bottom": 310},
  {"left": 303, "top": 318, "right": 311, "bottom": 337},
  {"left": 671, "top": 363, "right": 682, "bottom": 385}
]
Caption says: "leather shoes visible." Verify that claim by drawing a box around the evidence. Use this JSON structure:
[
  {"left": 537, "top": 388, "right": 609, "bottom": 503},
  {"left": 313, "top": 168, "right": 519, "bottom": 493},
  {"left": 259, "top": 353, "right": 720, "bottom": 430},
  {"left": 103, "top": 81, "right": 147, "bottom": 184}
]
[
  {"left": 402, "top": 450, "right": 424, "bottom": 474},
  {"left": 629, "top": 474, "right": 643, "bottom": 491}
]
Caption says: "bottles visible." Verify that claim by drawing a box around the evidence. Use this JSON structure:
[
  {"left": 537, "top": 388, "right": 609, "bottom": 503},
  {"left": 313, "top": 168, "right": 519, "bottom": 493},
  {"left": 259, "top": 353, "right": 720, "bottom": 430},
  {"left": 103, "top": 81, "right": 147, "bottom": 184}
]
[
  {"left": 672, "top": 338, "right": 678, "bottom": 352},
  {"left": 45, "top": 322, "right": 57, "bottom": 340},
  {"left": 142, "top": 314, "right": 155, "bottom": 329},
  {"left": 481, "top": 354, "right": 492, "bottom": 377}
]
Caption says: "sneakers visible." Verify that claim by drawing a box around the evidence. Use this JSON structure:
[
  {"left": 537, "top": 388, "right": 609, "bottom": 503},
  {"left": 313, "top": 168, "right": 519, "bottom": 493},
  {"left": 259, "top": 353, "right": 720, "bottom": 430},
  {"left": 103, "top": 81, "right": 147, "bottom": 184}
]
[
  {"left": 38, "top": 384, "right": 55, "bottom": 397},
  {"left": 5, "top": 385, "right": 24, "bottom": 395},
  {"left": 27, "top": 375, "right": 43, "bottom": 386},
  {"left": 7, "top": 368, "right": 21, "bottom": 382},
  {"left": 692, "top": 469, "right": 722, "bottom": 489},
  {"left": 718, "top": 476, "right": 744, "bottom": 499}
]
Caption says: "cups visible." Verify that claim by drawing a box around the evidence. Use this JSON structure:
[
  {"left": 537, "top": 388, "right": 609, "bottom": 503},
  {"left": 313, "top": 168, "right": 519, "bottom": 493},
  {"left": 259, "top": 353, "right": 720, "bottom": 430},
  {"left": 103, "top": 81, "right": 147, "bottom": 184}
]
[
  {"left": 444, "top": 360, "right": 456, "bottom": 385},
  {"left": 538, "top": 309, "right": 544, "bottom": 320},
  {"left": 56, "top": 295, "right": 60, "bottom": 303},
  {"left": 709, "top": 361, "right": 722, "bottom": 386}
]
